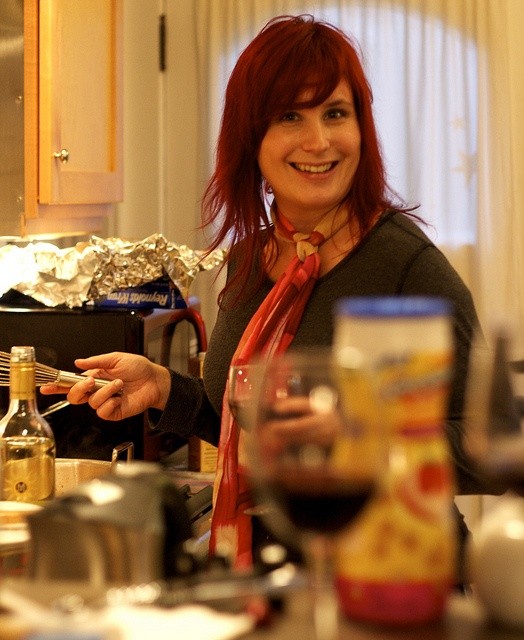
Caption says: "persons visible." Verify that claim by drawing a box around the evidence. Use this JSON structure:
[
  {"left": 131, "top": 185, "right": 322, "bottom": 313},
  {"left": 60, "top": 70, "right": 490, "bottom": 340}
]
[{"left": 39, "top": 13, "right": 494, "bottom": 623}]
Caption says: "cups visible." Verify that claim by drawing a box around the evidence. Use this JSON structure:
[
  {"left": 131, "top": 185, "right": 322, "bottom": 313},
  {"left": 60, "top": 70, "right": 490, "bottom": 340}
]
[{"left": 0, "top": 346, "right": 56, "bottom": 501}]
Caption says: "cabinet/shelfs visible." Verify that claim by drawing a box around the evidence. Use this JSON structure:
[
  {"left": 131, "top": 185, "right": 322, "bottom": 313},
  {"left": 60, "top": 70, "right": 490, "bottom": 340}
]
[
  {"left": 1, "top": 297, "right": 208, "bottom": 471},
  {"left": 22, "top": 0, "right": 127, "bottom": 221}
]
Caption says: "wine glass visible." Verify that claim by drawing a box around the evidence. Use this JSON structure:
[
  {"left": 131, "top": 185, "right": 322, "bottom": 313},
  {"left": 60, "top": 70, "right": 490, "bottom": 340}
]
[
  {"left": 256, "top": 363, "right": 385, "bottom": 640},
  {"left": 228, "top": 365, "right": 301, "bottom": 516}
]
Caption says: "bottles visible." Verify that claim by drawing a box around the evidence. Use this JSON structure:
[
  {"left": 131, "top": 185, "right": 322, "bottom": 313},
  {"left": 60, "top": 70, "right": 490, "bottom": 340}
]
[{"left": 332, "top": 296, "right": 457, "bottom": 628}]
